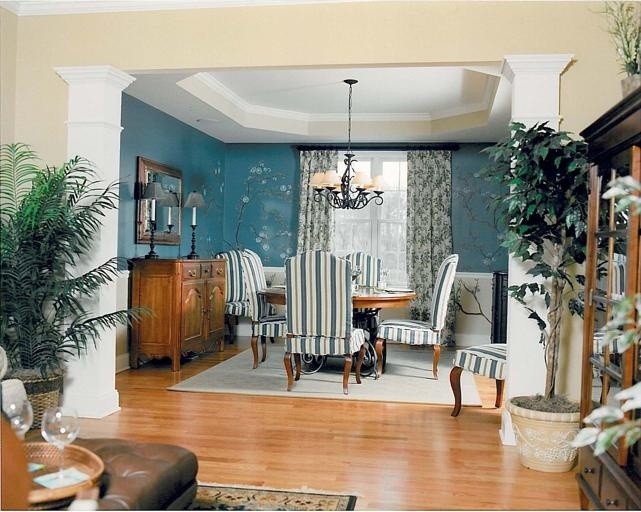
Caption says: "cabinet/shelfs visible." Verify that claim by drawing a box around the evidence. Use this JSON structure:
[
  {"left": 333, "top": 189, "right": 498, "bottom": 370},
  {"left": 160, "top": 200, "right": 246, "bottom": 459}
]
[
  {"left": 128, "top": 255, "right": 229, "bottom": 371},
  {"left": 575, "top": 84, "right": 641, "bottom": 511}
]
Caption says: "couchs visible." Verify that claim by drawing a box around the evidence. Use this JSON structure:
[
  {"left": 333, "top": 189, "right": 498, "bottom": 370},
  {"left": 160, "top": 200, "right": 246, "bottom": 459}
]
[{"left": 21, "top": 431, "right": 206, "bottom": 510}]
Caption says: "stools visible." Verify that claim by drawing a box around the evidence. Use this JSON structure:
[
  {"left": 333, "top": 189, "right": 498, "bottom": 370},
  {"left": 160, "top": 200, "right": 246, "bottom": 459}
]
[{"left": 449, "top": 341, "right": 508, "bottom": 416}]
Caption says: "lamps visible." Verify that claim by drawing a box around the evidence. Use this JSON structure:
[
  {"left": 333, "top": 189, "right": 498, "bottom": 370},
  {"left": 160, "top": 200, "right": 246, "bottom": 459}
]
[
  {"left": 141, "top": 182, "right": 165, "bottom": 260},
  {"left": 309, "top": 77, "right": 386, "bottom": 210},
  {"left": 184, "top": 190, "right": 206, "bottom": 257},
  {"left": 158, "top": 191, "right": 180, "bottom": 233}
]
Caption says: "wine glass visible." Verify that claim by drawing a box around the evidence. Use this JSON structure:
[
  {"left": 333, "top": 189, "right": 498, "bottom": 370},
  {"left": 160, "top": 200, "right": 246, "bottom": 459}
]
[{"left": 42, "top": 407, "right": 79, "bottom": 483}]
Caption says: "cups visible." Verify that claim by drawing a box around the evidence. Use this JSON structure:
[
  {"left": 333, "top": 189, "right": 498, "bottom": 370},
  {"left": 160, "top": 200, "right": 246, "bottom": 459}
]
[
  {"left": 1, "top": 379, "right": 34, "bottom": 439},
  {"left": 377, "top": 281, "right": 387, "bottom": 289}
]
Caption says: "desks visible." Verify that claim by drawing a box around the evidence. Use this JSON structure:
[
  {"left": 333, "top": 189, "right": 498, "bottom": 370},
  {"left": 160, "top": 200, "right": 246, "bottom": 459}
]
[{"left": 257, "top": 284, "right": 418, "bottom": 371}]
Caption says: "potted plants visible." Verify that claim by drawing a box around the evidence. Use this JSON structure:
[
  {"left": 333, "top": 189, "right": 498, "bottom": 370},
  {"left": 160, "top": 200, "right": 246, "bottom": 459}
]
[
  {"left": 0, "top": 143, "right": 154, "bottom": 428},
  {"left": 474, "top": 117, "right": 594, "bottom": 474},
  {"left": 589, "top": 0, "right": 641, "bottom": 98}
]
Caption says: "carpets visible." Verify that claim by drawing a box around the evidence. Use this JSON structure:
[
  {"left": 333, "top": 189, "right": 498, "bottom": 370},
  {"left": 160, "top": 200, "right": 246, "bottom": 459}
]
[
  {"left": 184, "top": 480, "right": 359, "bottom": 511},
  {"left": 167, "top": 341, "right": 482, "bottom": 412}
]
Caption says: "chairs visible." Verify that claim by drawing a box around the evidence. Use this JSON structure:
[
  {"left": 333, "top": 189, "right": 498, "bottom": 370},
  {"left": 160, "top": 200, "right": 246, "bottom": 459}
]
[
  {"left": 282, "top": 250, "right": 371, "bottom": 394},
  {"left": 215, "top": 247, "right": 278, "bottom": 343},
  {"left": 375, "top": 251, "right": 462, "bottom": 381},
  {"left": 338, "top": 250, "right": 387, "bottom": 369},
  {"left": 238, "top": 248, "right": 290, "bottom": 370}
]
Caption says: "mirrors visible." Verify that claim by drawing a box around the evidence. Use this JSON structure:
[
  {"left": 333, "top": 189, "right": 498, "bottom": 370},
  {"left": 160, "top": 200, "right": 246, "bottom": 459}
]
[{"left": 135, "top": 155, "right": 183, "bottom": 247}]
